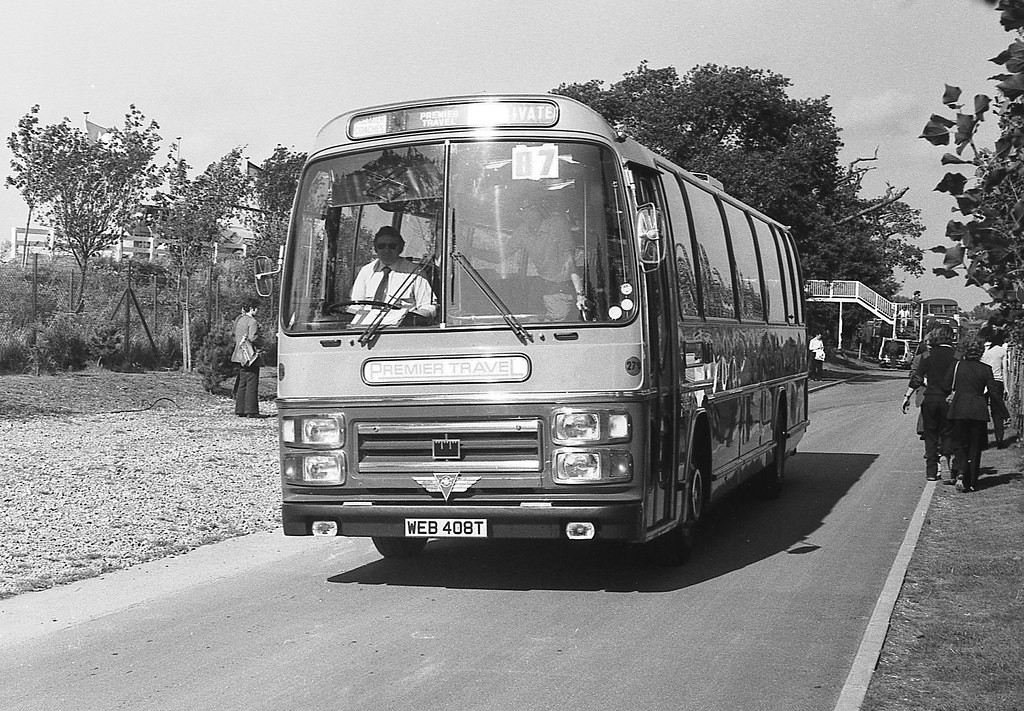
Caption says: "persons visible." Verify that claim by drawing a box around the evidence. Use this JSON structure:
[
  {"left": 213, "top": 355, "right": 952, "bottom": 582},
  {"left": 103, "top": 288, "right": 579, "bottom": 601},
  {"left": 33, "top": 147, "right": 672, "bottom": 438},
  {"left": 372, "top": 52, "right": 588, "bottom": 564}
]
[
  {"left": 901, "top": 324, "right": 1010, "bottom": 492},
  {"left": 462, "top": 195, "right": 592, "bottom": 323},
  {"left": 887, "top": 339, "right": 898, "bottom": 371},
  {"left": 683, "top": 342, "right": 809, "bottom": 448},
  {"left": 343, "top": 225, "right": 437, "bottom": 331},
  {"left": 913, "top": 308, "right": 920, "bottom": 333},
  {"left": 231, "top": 298, "right": 266, "bottom": 419},
  {"left": 897, "top": 305, "right": 911, "bottom": 334},
  {"left": 809, "top": 331, "right": 825, "bottom": 382}
]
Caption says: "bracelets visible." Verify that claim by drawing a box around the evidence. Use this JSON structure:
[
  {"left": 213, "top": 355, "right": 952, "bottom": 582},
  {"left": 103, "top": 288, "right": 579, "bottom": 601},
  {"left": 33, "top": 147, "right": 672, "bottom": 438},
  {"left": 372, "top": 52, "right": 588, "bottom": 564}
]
[{"left": 904, "top": 394, "right": 910, "bottom": 400}]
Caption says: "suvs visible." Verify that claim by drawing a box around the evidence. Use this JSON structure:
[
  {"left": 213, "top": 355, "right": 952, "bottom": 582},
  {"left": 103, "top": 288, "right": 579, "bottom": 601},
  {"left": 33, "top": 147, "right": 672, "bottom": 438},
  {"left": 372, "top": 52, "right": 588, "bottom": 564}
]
[{"left": 878, "top": 336, "right": 914, "bottom": 370}]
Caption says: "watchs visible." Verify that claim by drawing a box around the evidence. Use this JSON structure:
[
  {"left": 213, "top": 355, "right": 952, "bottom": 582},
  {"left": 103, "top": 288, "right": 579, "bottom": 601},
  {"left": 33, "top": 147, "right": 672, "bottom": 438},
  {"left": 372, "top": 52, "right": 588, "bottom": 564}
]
[{"left": 575, "top": 290, "right": 585, "bottom": 297}]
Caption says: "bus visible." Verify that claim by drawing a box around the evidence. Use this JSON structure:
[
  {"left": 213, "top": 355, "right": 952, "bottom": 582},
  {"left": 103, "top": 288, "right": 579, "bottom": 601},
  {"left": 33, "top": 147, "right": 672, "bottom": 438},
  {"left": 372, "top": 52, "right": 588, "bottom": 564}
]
[
  {"left": 919, "top": 298, "right": 962, "bottom": 351},
  {"left": 273, "top": 94, "right": 809, "bottom": 545}
]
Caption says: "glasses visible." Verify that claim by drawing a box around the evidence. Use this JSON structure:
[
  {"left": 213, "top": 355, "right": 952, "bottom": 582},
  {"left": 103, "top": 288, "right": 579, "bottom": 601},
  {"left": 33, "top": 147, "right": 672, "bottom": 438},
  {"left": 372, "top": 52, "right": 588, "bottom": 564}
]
[{"left": 374, "top": 243, "right": 399, "bottom": 250}]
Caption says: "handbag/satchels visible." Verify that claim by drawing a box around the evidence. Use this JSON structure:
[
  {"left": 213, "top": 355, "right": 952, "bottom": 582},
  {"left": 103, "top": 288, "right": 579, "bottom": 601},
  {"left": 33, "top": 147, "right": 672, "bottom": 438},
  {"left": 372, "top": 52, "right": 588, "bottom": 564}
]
[
  {"left": 946, "top": 360, "right": 960, "bottom": 404},
  {"left": 815, "top": 348, "right": 825, "bottom": 362},
  {"left": 238, "top": 336, "right": 260, "bottom": 368}
]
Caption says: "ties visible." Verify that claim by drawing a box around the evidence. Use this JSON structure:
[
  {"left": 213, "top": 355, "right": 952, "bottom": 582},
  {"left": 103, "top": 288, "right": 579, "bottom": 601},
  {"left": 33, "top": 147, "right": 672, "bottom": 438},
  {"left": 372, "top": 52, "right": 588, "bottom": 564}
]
[{"left": 372, "top": 266, "right": 392, "bottom": 309}]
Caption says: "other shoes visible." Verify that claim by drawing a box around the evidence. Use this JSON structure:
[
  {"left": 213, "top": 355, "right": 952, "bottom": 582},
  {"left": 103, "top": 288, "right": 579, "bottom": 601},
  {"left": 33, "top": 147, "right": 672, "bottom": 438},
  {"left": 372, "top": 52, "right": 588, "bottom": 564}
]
[
  {"left": 955, "top": 474, "right": 962, "bottom": 491},
  {"left": 939, "top": 456, "right": 952, "bottom": 482},
  {"left": 926, "top": 473, "right": 936, "bottom": 481},
  {"left": 247, "top": 412, "right": 262, "bottom": 418},
  {"left": 238, "top": 412, "right": 247, "bottom": 417}
]
